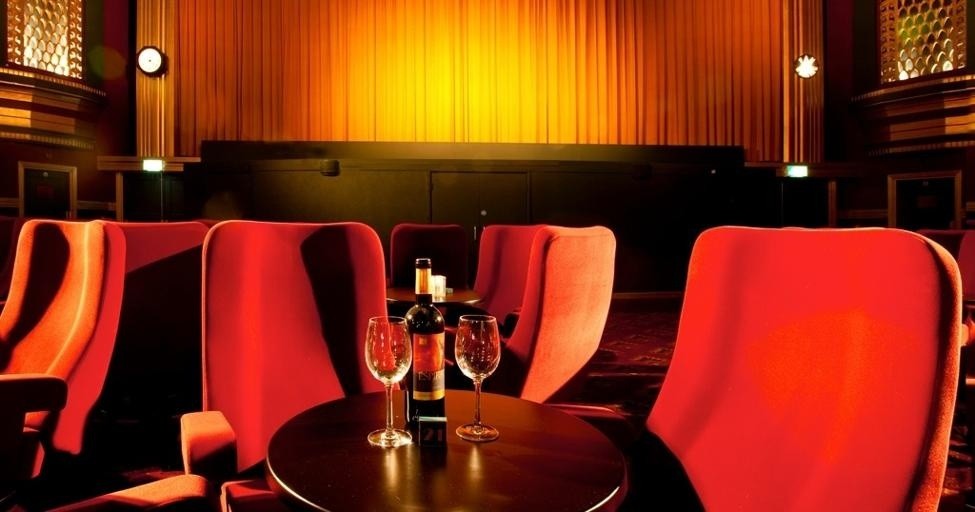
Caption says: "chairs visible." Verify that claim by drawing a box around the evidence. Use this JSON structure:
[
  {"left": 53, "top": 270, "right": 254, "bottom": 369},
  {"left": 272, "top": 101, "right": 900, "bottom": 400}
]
[
  {"left": 632, "top": 226, "right": 963, "bottom": 512},
  {"left": 383, "top": 221, "right": 617, "bottom": 410},
  {"left": 180, "top": 221, "right": 396, "bottom": 512},
  {"left": 0, "top": 219, "right": 216, "bottom": 508}
]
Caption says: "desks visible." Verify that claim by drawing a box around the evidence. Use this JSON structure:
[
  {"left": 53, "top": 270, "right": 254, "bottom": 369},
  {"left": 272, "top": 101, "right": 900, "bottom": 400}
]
[{"left": 266, "top": 389, "right": 629, "bottom": 512}]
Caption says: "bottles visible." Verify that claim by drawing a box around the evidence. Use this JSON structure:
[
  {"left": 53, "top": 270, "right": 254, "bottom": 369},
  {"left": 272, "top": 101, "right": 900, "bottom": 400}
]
[{"left": 401, "top": 257, "right": 448, "bottom": 453}]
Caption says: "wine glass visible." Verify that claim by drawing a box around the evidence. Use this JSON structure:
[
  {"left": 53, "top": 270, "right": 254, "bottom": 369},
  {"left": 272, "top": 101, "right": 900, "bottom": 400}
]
[
  {"left": 455, "top": 314, "right": 500, "bottom": 444},
  {"left": 364, "top": 316, "right": 413, "bottom": 447}
]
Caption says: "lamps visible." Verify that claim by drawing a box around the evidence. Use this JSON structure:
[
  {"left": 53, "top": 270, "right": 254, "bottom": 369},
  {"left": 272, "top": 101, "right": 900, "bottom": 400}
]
[{"left": 793, "top": 53, "right": 819, "bottom": 80}]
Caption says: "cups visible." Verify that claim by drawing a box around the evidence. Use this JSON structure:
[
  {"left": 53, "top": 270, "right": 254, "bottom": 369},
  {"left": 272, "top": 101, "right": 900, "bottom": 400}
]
[{"left": 431, "top": 275, "right": 447, "bottom": 304}]
